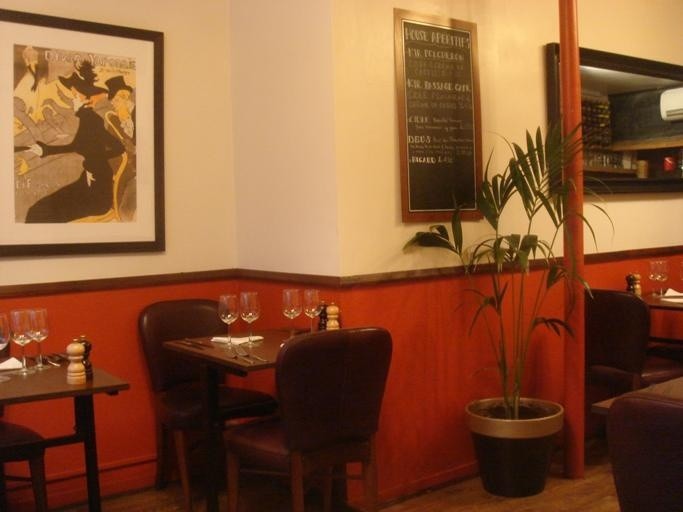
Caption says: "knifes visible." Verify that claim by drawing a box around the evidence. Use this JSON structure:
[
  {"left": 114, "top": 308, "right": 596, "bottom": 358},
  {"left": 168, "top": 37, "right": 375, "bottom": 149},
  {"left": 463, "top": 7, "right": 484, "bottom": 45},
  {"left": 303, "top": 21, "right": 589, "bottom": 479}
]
[{"left": 176, "top": 338, "right": 214, "bottom": 352}]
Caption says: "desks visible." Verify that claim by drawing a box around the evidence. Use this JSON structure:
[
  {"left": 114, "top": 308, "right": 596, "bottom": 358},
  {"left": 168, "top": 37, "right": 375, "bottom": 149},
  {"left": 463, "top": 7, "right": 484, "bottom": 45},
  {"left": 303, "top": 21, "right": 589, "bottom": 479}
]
[{"left": 139, "top": 299, "right": 281, "bottom": 512}]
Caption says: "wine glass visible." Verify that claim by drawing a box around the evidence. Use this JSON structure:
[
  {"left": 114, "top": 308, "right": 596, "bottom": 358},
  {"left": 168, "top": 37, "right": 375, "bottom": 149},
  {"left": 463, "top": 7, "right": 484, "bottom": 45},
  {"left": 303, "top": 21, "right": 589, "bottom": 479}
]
[
  {"left": 282, "top": 288, "right": 321, "bottom": 335},
  {"left": 218, "top": 291, "right": 261, "bottom": 350},
  {"left": 649, "top": 261, "right": 669, "bottom": 297},
  {"left": 0, "top": 308, "right": 49, "bottom": 383}
]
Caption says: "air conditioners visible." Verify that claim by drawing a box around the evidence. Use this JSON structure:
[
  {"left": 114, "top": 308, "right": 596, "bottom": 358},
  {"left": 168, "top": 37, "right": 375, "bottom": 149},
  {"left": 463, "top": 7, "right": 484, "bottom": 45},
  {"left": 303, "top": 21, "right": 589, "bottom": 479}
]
[{"left": 659, "top": 87, "right": 683, "bottom": 123}]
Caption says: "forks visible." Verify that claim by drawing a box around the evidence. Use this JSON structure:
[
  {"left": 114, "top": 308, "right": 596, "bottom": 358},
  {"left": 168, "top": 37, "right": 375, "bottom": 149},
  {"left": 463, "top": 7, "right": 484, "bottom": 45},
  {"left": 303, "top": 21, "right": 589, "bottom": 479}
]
[{"left": 226, "top": 344, "right": 269, "bottom": 363}]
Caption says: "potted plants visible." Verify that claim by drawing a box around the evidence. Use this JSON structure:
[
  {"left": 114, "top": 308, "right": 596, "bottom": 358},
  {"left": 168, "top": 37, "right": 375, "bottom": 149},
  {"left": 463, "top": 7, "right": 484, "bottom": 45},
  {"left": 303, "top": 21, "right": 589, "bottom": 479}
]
[{"left": 402, "top": 117, "right": 618, "bottom": 500}]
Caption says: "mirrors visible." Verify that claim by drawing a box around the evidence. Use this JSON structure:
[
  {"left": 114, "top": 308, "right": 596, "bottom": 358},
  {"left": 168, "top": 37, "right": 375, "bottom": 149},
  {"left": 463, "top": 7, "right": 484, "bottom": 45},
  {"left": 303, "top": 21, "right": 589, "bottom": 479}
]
[{"left": 542, "top": 41, "right": 682, "bottom": 198}]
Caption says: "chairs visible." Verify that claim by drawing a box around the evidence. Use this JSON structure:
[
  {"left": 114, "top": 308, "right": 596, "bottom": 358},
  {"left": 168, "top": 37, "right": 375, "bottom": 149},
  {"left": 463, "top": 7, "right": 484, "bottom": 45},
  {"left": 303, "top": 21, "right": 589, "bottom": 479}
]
[
  {"left": 0, "top": 328, "right": 49, "bottom": 512},
  {"left": 584, "top": 284, "right": 683, "bottom": 453},
  {"left": 226, "top": 326, "right": 393, "bottom": 511},
  {"left": 164, "top": 333, "right": 292, "bottom": 511}
]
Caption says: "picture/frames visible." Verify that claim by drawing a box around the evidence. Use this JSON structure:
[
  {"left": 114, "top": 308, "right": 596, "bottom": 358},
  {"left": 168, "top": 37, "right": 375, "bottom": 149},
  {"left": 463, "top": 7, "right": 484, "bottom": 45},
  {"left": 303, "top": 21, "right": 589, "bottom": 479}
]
[
  {"left": 0, "top": 9, "right": 167, "bottom": 260},
  {"left": 391, "top": 6, "right": 487, "bottom": 225}
]
